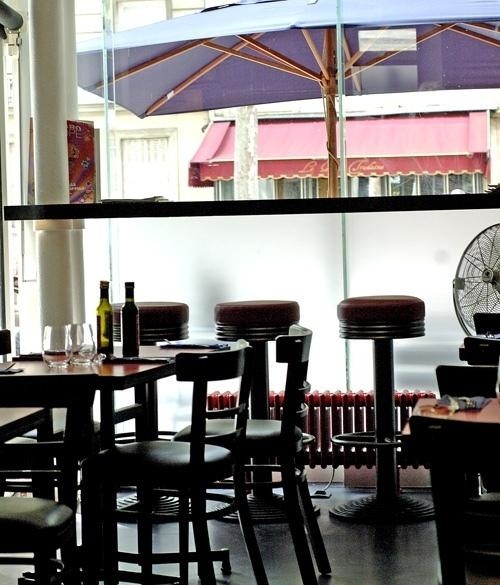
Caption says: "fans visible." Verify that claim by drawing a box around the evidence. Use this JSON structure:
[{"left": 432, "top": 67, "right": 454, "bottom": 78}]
[{"left": 454, "top": 224, "right": 500, "bottom": 339}]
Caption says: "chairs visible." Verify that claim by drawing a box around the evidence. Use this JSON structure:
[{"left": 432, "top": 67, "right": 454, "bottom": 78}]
[{"left": 408, "top": 364, "right": 500, "bottom": 585}]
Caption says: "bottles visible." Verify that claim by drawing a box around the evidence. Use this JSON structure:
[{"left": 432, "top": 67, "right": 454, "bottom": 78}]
[
  {"left": 95, "top": 281, "right": 114, "bottom": 355},
  {"left": 120, "top": 281, "right": 141, "bottom": 357}
]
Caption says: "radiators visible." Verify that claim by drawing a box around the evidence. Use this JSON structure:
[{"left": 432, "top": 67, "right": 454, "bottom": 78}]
[{"left": 206, "top": 390, "right": 435, "bottom": 470}]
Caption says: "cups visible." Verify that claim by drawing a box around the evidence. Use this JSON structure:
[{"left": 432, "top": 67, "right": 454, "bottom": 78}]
[
  {"left": 65, "top": 322, "right": 96, "bottom": 364},
  {"left": 41, "top": 324, "right": 72, "bottom": 367}
]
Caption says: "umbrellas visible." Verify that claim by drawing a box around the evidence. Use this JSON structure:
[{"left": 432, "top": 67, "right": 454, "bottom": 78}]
[{"left": 75, "top": 0, "right": 500, "bottom": 198}]
